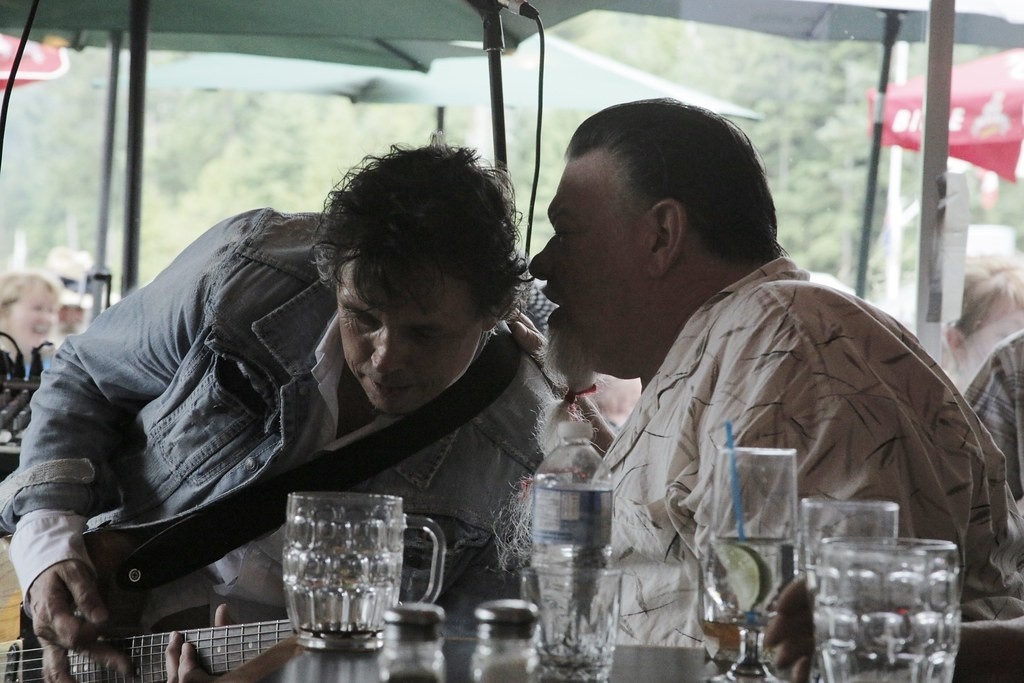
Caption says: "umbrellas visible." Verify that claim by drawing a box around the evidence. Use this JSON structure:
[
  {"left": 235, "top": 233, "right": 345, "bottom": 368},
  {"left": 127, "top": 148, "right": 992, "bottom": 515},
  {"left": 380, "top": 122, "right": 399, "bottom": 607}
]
[{"left": 0, "top": 0, "right": 1024, "bottom": 322}]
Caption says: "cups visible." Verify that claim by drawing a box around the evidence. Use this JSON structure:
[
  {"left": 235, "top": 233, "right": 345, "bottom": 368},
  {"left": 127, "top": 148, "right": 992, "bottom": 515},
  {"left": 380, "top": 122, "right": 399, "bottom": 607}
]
[
  {"left": 283, "top": 491, "right": 447, "bottom": 649},
  {"left": 522, "top": 567, "right": 622, "bottom": 683},
  {"left": 817, "top": 537, "right": 959, "bottom": 683}
]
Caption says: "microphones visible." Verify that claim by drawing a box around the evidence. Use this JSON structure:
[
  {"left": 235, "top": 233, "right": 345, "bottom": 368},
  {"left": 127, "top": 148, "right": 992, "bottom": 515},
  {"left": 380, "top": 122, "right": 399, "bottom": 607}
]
[{"left": 91, "top": 268, "right": 112, "bottom": 282}]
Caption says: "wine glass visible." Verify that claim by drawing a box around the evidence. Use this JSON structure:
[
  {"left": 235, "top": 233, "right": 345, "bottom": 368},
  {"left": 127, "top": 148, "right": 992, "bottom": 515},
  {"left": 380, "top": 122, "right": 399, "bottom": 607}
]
[
  {"left": 705, "top": 447, "right": 800, "bottom": 683},
  {"left": 801, "top": 498, "right": 900, "bottom": 683}
]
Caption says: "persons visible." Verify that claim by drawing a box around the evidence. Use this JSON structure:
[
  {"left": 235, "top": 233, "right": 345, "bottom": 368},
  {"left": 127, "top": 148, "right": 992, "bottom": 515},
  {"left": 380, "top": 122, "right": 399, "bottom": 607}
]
[
  {"left": 0, "top": 144, "right": 576, "bottom": 682},
  {"left": 525, "top": 97, "right": 1024, "bottom": 683}
]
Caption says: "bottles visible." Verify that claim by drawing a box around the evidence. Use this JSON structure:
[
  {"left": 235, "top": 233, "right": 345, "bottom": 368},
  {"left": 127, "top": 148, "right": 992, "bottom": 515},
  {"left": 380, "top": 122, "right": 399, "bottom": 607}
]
[
  {"left": 378, "top": 604, "right": 446, "bottom": 683},
  {"left": 470, "top": 598, "right": 541, "bottom": 683},
  {"left": 530, "top": 422, "right": 614, "bottom": 598}
]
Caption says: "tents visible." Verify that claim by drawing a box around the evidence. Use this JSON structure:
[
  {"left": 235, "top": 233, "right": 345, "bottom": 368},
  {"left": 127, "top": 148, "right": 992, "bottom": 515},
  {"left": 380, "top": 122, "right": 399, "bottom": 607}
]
[{"left": 867, "top": 49, "right": 1024, "bottom": 182}]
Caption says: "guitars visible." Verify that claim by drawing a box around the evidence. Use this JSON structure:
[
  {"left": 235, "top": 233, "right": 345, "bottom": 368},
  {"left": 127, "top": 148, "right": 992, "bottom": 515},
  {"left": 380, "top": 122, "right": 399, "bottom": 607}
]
[{"left": 0, "top": 503, "right": 380, "bottom": 683}]
